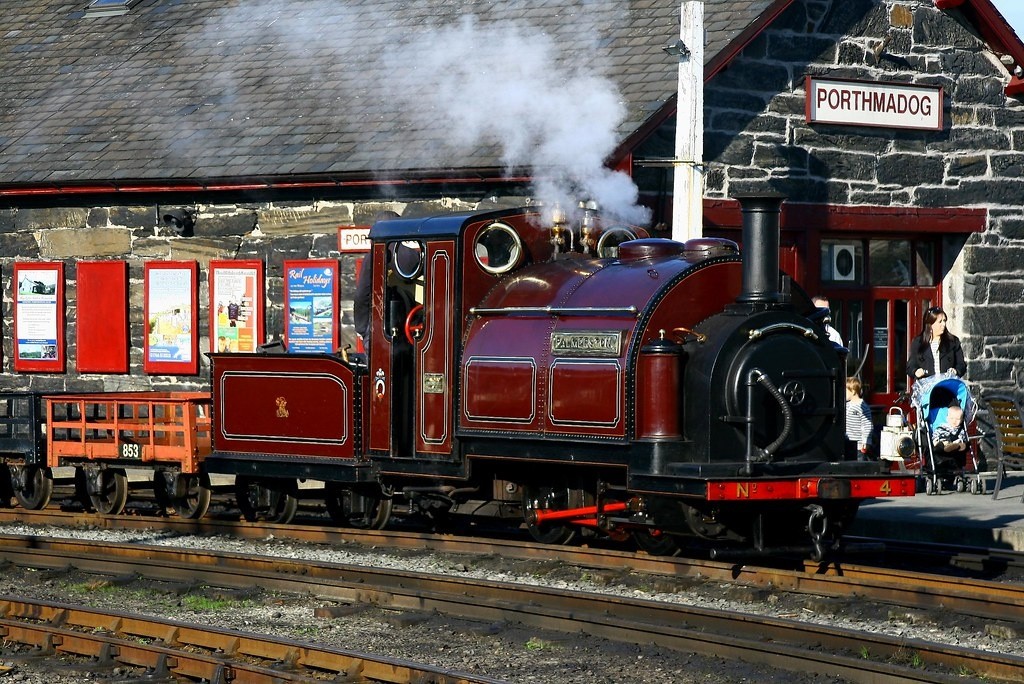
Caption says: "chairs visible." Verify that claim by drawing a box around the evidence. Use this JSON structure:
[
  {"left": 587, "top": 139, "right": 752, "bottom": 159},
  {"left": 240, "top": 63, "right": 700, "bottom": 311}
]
[{"left": 980, "top": 394, "right": 1024, "bottom": 503}]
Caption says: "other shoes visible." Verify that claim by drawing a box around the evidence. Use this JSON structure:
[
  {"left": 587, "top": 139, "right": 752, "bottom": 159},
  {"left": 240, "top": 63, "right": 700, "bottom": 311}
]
[
  {"left": 944, "top": 443, "right": 959, "bottom": 452},
  {"left": 957, "top": 442, "right": 966, "bottom": 451}
]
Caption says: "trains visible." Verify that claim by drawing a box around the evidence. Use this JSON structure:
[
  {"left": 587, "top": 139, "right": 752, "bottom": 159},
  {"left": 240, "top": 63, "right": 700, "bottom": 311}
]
[{"left": 0, "top": 194, "right": 915, "bottom": 561}]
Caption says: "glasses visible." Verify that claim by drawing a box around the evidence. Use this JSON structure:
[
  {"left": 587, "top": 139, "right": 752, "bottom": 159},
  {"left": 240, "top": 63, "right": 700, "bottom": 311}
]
[{"left": 928, "top": 306, "right": 941, "bottom": 317}]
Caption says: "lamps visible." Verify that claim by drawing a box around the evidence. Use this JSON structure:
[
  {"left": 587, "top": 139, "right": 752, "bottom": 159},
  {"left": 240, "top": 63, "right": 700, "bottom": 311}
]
[{"left": 661, "top": 38, "right": 687, "bottom": 58}]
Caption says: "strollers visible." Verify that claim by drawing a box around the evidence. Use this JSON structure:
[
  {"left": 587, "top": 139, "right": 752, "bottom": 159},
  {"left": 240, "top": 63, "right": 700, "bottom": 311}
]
[{"left": 910, "top": 369, "right": 986, "bottom": 495}]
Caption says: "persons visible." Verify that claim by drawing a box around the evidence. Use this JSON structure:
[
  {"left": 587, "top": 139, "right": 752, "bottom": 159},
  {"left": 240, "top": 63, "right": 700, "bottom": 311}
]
[
  {"left": 846, "top": 377, "right": 872, "bottom": 460},
  {"left": 905, "top": 307, "right": 967, "bottom": 378},
  {"left": 218, "top": 295, "right": 245, "bottom": 327},
  {"left": 812, "top": 296, "right": 843, "bottom": 348},
  {"left": 353, "top": 242, "right": 423, "bottom": 369},
  {"left": 218, "top": 336, "right": 238, "bottom": 353},
  {"left": 931, "top": 405, "right": 967, "bottom": 452}
]
